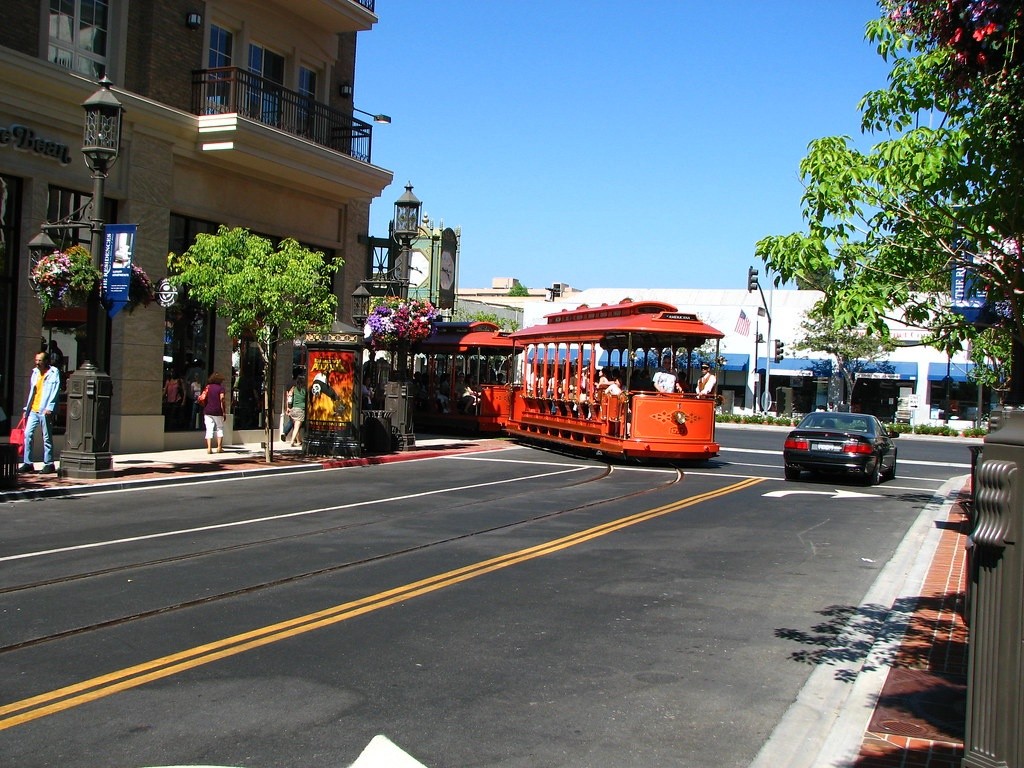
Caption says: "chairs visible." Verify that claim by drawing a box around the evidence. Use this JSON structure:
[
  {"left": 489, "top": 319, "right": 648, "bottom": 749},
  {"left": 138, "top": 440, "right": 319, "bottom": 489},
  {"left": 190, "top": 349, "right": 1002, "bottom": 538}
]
[{"left": 413, "top": 373, "right": 625, "bottom": 425}]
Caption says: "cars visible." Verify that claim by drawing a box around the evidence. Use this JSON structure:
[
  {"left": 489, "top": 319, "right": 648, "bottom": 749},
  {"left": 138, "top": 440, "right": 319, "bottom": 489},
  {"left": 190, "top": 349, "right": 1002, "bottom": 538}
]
[{"left": 782, "top": 411, "right": 900, "bottom": 486}]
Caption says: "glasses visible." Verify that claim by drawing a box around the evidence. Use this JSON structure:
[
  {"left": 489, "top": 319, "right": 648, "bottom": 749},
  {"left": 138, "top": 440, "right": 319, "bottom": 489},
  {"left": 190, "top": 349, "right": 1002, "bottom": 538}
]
[
  {"left": 700, "top": 366, "right": 709, "bottom": 371},
  {"left": 663, "top": 361, "right": 671, "bottom": 365}
]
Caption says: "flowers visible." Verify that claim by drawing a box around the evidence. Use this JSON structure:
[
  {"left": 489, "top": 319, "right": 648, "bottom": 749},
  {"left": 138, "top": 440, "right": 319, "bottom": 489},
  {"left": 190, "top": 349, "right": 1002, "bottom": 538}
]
[
  {"left": 30, "top": 248, "right": 158, "bottom": 315},
  {"left": 365, "top": 293, "right": 441, "bottom": 349}
]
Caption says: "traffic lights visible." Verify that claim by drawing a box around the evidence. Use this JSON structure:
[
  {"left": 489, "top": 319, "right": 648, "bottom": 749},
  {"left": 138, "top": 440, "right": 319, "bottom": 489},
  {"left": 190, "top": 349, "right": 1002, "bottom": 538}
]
[
  {"left": 774, "top": 339, "right": 784, "bottom": 363},
  {"left": 747, "top": 265, "right": 759, "bottom": 293}
]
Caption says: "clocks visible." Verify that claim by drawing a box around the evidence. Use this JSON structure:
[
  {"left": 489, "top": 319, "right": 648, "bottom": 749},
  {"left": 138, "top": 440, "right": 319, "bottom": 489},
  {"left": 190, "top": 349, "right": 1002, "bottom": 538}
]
[
  {"left": 440, "top": 251, "right": 455, "bottom": 289},
  {"left": 394, "top": 246, "right": 431, "bottom": 288}
]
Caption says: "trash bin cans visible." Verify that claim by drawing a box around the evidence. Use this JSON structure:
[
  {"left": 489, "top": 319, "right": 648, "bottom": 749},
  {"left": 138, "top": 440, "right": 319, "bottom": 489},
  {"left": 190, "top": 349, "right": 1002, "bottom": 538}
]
[
  {"left": 0, "top": 442, "right": 21, "bottom": 490},
  {"left": 966, "top": 444, "right": 984, "bottom": 497},
  {"left": 361, "top": 408, "right": 398, "bottom": 452}
]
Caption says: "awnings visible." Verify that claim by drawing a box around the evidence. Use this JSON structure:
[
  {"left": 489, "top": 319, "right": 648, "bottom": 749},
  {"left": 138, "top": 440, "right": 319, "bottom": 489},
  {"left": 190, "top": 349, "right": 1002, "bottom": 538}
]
[
  {"left": 598, "top": 350, "right": 660, "bottom": 368},
  {"left": 756, "top": 357, "right": 833, "bottom": 378},
  {"left": 527, "top": 348, "right": 591, "bottom": 367},
  {"left": 927, "top": 362, "right": 1003, "bottom": 384},
  {"left": 674, "top": 351, "right": 750, "bottom": 372},
  {"left": 838, "top": 359, "right": 918, "bottom": 383}
]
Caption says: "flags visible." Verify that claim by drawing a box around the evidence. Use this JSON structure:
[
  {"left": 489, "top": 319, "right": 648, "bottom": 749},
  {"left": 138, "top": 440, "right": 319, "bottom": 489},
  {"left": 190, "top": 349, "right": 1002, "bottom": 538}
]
[{"left": 734, "top": 309, "right": 751, "bottom": 337}]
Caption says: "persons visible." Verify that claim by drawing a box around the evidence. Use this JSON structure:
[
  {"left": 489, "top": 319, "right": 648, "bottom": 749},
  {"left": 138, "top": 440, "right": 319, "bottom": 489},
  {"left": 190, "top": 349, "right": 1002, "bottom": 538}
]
[
  {"left": 632, "top": 355, "right": 716, "bottom": 400},
  {"left": 409, "top": 371, "right": 505, "bottom": 415},
  {"left": 197, "top": 371, "right": 228, "bottom": 454},
  {"left": 527, "top": 365, "right": 631, "bottom": 437},
  {"left": 362, "top": 376, "right": 377, "bottom": 410},
  {"left": 280, "top": 374, "right": 305, "bottom": 446},
  {"left": 189, "top": 370, "right": 201, "bottom": 429},
  {"left": 16, "top": 352, "right": 61, "bottom": 475},
  {"left": 161, "top": 366, "right": 186, "bottom": 432}
]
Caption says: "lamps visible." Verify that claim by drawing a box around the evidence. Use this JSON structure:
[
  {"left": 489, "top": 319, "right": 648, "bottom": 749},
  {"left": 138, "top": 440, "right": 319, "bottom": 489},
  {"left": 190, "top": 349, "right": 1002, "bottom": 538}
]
[
  {"left": 188, "top": 8, "right": 202, "bottom": 31},
  {"left": 339, "top": 81, "right": 352, "bottom": 99},
  {"left": 353, "top": 109, "right": 392, "bottom": 124}
]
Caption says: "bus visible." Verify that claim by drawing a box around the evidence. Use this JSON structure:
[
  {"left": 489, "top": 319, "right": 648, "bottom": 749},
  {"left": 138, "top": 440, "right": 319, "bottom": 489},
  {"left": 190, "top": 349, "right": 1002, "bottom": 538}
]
[
  {"left": 362, "top": 320, "right": 526, "bottom": 437},
  {"left": 501, "top": 297, "right": 728, "bottom": 468}
]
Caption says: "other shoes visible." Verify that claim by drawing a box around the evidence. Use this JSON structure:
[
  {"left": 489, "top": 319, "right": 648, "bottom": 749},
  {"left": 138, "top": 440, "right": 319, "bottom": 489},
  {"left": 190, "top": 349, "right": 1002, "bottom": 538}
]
[
  {"left": 217, "top": 449, "right": 224, "bottom": 452},
  {"left": 296, "top": 442, "right": 303, "bottom": 445},
  {"left": 291, "top": 443, "right": 295, "bottom": 447},
  {"left": 208, "top": 450, "right": 213, "bottom": 454},
  {"left": 16, "top": 463, "right": 34, "bottom": 473},
  {"left": 281, "top": 433, "right": 286, "bottom": 442},
  {"left": 38, "top": 464, "right": 55, "bottom": 474}
]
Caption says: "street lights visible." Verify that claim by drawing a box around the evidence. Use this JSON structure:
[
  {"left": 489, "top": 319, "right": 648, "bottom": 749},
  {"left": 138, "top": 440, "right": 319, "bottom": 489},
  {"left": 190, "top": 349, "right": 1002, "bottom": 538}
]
[
  {"left": 387, "top": 178, "right": 423, "bottom": 452},
  {"left": 26, "top": 70, "right": 125, "bottom": 480},
  {"left": 340, "top": 284, "right": 371, "bottom": 459}
]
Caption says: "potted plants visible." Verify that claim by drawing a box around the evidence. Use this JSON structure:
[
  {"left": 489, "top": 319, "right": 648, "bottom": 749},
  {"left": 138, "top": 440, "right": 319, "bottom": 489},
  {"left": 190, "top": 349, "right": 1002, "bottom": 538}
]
[{"left": 715, "top": 412, "right": 990, "bottom": 439}]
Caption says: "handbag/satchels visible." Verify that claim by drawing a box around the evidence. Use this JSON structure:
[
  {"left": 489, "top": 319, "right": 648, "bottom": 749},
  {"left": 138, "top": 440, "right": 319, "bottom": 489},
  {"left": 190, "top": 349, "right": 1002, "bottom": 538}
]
[
  {"left": 198, "top": 384, "right": 209, "bottom": 407},
  {"left": 287, "top": 385, "right": 297, "bottom": 409},
  {"left": 9, "top": 419, "right": 26, "bottom": 445}
]
